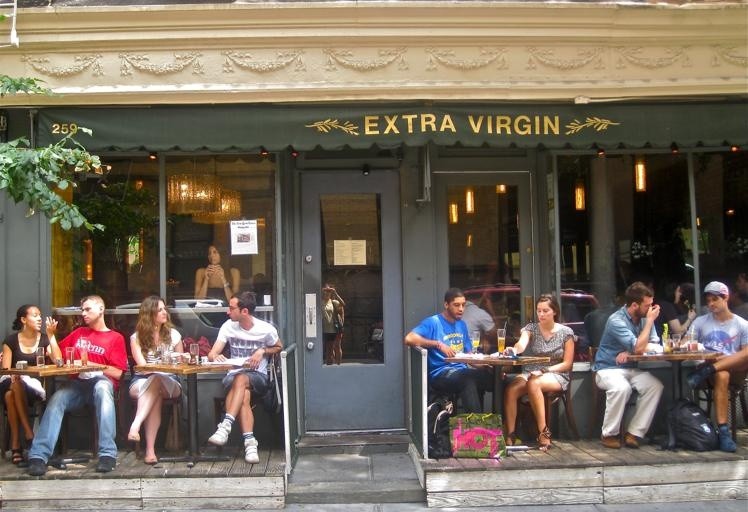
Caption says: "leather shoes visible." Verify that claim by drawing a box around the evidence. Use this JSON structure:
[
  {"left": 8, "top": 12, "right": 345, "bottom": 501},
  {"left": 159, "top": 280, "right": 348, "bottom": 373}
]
[
  {"left": 145, "top": 456, "right": 158, "bottom": 464},
  {"left": 601, "top": 432, "right": 638, "bottom": 448},
  {"left": 128, "top": 433, "right": 140, "bottom": 441}
]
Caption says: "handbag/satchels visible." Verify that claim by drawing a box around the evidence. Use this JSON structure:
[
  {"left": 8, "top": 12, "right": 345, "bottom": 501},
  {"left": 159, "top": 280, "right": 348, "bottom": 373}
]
[
  {"left": 332, "top": 300, "right": 344, "bottom": 328},
  {"left": 428, "top": 402, "right": 506, "bottom": 458}
]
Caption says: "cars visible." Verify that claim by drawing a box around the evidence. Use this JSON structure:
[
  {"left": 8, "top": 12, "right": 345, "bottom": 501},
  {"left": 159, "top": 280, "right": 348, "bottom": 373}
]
[{"left": 110, "top": 301, "right": 225, "bottom": 356}]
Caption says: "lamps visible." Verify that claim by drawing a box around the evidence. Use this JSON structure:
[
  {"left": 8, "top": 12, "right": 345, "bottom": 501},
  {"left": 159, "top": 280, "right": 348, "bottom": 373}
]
[
  {"left": 167, "top": 155, "right": 241, "bottom": 226},
  {"left": 148, "top": 146, "right": 300, "bottom": 162},
  {"left": 597, "top": 142, "right": 741, "bottom": 156},
  {"left": 449, "top": 153, "right": 646, "bottom": 225}
]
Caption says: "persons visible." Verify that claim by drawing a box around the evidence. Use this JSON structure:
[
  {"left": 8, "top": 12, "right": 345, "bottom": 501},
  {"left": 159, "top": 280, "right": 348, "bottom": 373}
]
[
  {"left": 319, "top": 280, "right": 345, "bottom": 364},
  {"left": 736, "top": 272, "right": 748, "bottom": 295},
  {"left": 207, "top": 291, "right": 282, "bottom": 465},
  {"left": 502, "top": 293, "right": 575, "bottom": 452},
  {"left": 26, "top": 294, "right": 128, "bottom": 478},
  {"left": 651, "top": 269, "right": 696, "bottom": 344},
  {"left": 332, "top": 310, "right": 344, "bottom": 365},
  {"left": 0, "top": 304, "right": 63, "bottom": 464},
  {"left": 127, "top": 295, "right": 186, "bottom": 466},
  {"left": 727, "top": 285, "right": 748, "bottom": 321},
  {"left": 680, "top": 281, "right": 747, "bottom": 453},
  {"left": 560, "top": 296, "right": 589, "bottom": 355},
  {"left": 403, "top": 287, "right": 517, "bottom": 413},
  {"left": 581, "top": 283, "right": 624, "bottom": 346},
  {"left": 591, "top": 281, "right": 664, "bottom": 449},
  {"left": 461, "top": 279, "right": 499, "bottom": 354},
  {"left": 193, "top": 241, "right": 241, "bottom": 326}
]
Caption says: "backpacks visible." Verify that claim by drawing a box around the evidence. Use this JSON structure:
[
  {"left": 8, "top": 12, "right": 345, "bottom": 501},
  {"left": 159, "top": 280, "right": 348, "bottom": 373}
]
[{"left": 666, "top": 398, "right": 720, "bottom": 451}]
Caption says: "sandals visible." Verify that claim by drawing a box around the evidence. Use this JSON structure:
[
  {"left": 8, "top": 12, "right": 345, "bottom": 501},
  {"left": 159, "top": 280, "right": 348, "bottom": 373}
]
[
  {"left": 12, "top": 449, "right": 23, "bottom": 463},
  {"left": 536, "top": 427, "right": 552, "bottom": 451}
]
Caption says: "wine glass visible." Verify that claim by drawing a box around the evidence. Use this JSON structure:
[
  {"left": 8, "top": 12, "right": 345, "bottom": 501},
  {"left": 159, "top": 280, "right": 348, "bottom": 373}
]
[{"left": 471, "top": 329, "right": 481, "bottom": 354}]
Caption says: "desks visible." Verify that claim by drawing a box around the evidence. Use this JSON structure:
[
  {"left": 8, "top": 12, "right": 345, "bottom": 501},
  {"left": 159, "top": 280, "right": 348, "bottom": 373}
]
[
  {"left": 626, "top": 351, "right": 725, "bottom": 445},
  {"left": 443, "top": 353, "right": 551, "bottom": 446},
  {"left": 51, "top": 306, "right": 274, "bottom": 331}
]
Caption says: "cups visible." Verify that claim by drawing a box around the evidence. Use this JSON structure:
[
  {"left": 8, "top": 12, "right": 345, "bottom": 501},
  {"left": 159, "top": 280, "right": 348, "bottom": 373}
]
[
  {"left": 190, "top": 344, "right": 199, "bottom": 365},
  {"left": 56, "top": 359, "right": 64, "bottom": 368},
  {"left": 175, "top": 355, "right": 183, "bottom": 365},
  {"left": 36, "top": 347, "right": 45, "bottom": 368},
  {"left": 80, "top": 351, "right": 88, "bottom": 367},
  {"left": 663, "top": 339, "right": 671, "bottom": 353},
  {"left": 497, "top": 328, "right": 506, "bottom": 357},
  {"left": 672, "top": 333, "right": 681, "bottom": 350},
  {"left": 687, "top": 331, "right": 699, "bottom": 352},
  {"left": 160, "top": 342, "right": 171, "bottom": 365},
  {"left": 661, "top": 334, "right": 670, "bottom": 347},
  {"left": 65, "top": 347, "right": 75, "bottom": 367}
]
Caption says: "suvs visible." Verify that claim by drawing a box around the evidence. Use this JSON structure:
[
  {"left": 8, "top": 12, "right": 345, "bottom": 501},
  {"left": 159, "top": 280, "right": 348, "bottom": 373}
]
[{"left": 362, "top": 284, "right": 608, "bottom": 370}]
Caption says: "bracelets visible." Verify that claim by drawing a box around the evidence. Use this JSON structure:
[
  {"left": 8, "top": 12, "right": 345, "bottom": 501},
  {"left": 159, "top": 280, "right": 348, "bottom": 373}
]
[
  {"left": 436, "top": 341, "right": 442, "bottom": 350},
  {"left": 687, "top": 317, "right": 692, "bottom": 322},
  {"left": 541, "top": 366, "right": 549, "bottom": 373},
  {"left": 262, "top": 346, "right": 267, "bottom": 354},
  {"left": 223, "top": 282, "right": 229, "bottom": 288}
]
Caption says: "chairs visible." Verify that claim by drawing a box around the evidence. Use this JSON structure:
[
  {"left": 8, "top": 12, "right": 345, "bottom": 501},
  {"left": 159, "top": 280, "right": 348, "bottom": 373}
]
[
  {"left": 428, "top": 387, "right": 487, "bottom": 417},
  {"left": 690, "top": 376, "right": 747, "bottom": 445},
  {"left": 0, "top": 336, "right": 281, "bottom": 469},
  {"left": 516, "top": 335, "right": 580, "bottom": 444},
  {"left": 586, "top": 345, "right": 655, "bottom": 442}
]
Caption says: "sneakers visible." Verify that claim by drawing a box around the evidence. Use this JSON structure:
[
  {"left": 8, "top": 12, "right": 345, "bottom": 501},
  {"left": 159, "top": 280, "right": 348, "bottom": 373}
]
[
  {"left": 245, "top": 445, "right": 259, "bottom": 464},
  {"left": 96, "top": 456, "right": 116, "bottom": 471},
  {"left": 719, "top": 434, "right": 736, "bottom": 452},
  {"left": 208, "top": 428, "right": 228, "bottom": 445},
  {"left": 29, "top": 459, "right": 45, "bottom": 476},
  {"left": 687, "top": 371, "right": 702, "bottom": 389}
]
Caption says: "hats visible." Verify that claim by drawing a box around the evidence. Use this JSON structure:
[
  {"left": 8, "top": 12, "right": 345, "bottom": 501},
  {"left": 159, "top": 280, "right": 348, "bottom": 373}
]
[{"left": 701, "top": 281, "right": 729, "bottom": 295}]
